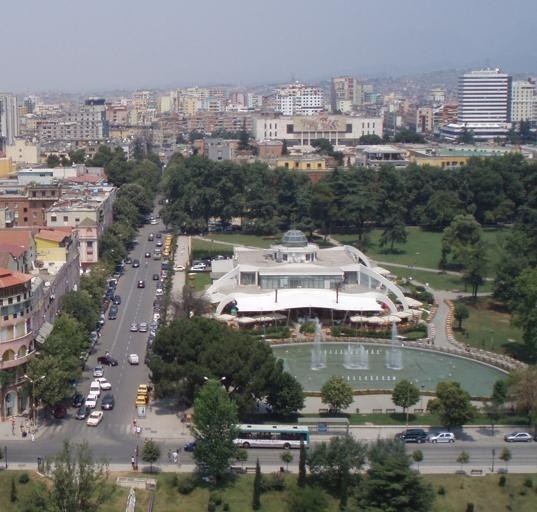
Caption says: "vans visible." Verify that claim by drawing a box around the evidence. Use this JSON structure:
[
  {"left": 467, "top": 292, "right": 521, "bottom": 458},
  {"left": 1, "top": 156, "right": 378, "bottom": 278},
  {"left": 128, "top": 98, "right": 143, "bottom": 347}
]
[{"left": 184, "top": 440, "right": 196, "bottom": 451}]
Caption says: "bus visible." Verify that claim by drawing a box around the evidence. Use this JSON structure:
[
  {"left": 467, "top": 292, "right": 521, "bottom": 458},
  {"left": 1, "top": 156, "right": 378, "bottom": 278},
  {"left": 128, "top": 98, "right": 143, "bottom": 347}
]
[{"left": 226, "top": 424, "right": 309, "bottom": 451}]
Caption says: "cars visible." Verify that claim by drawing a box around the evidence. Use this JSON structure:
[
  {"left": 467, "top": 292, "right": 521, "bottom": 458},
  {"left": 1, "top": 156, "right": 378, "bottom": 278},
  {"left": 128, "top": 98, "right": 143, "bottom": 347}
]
[
  {"left": 54, "top": 406, "right": 65, "bottom": 418},
  {"left": 135, "top": 383, "right": 150, "bottom": 396},
  {"left": 128, "top": 322, "right": 138, "bottom": 332},
  {"left": 138, "top": 322, "right": 148, "bottom": 332},
  {"left": 135, "top": 395, "right": 148, "bottom": 407},
  {"left": 429, "top": 432, "right": 455, "bottom": 443},
  {"left": 74, "top": 405, "right": 90, "bottom": 420},
  {"left": 532, "top": 432, "right": 536, "bottom": 441},
  {"left": 188, "top": 255, "right": 225, "bottom": 271},
  {"left": 68, "top": 323, "right": 119, "bottom": 410},
  {"left": 504, "top": 431, "right": 533, "bottom": 442},
  {"left": 132, "top": 198, "right": 175, "bottom": 288},
  {"left": 173, "top": 265, "right": 185, "bottom": 272},
  {"left": 184, "top": 221, "right": 240, "bottom": 232},
  {"left": 126, "top": 354, "right": 138, "bottom": 365},
  {"left": 86, "top": 410, "right": 103, "bottom": 427},
  {"left": 97, "top": 255, "right": 132, "bottom": 325},
  {"left": 145, "top": 288, "right": 164, "bottom": 365}
]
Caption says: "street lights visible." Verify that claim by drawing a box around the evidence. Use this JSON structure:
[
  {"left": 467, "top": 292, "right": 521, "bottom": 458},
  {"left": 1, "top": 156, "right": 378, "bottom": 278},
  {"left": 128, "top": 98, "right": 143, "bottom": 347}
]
[
  {"left": 489, "top": 329, "right": 494, "bottom": 350},
  {"left": 202, "top": 375, "right": 227, "bottom": 384},
  {"left": 419, "top": 384, "right": 423, "bottom": 410},
  {"left": 405, "top": 380, "right": 412, "bottom": 427},
  {"left": 23, "top": 374, "right": 46, "bottom": 426}
]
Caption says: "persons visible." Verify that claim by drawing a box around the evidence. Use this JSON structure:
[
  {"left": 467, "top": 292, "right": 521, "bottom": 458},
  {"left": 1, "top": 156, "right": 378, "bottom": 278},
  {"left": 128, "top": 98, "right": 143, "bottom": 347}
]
[
  {"left": 172, "top": 450, "right": 178, "bottom": 463},
  {"left": 131, "top": 456, "right": 135, "bottom": 471},
  {"left": 167, "top": 448, "right": 172, "bottom": 463}
]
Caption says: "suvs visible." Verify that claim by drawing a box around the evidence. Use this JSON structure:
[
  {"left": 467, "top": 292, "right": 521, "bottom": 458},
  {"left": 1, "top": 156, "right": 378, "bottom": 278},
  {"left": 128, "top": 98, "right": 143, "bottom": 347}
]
[{"left": 393, "top": 428, "right": 428, "bottom": 443}]
